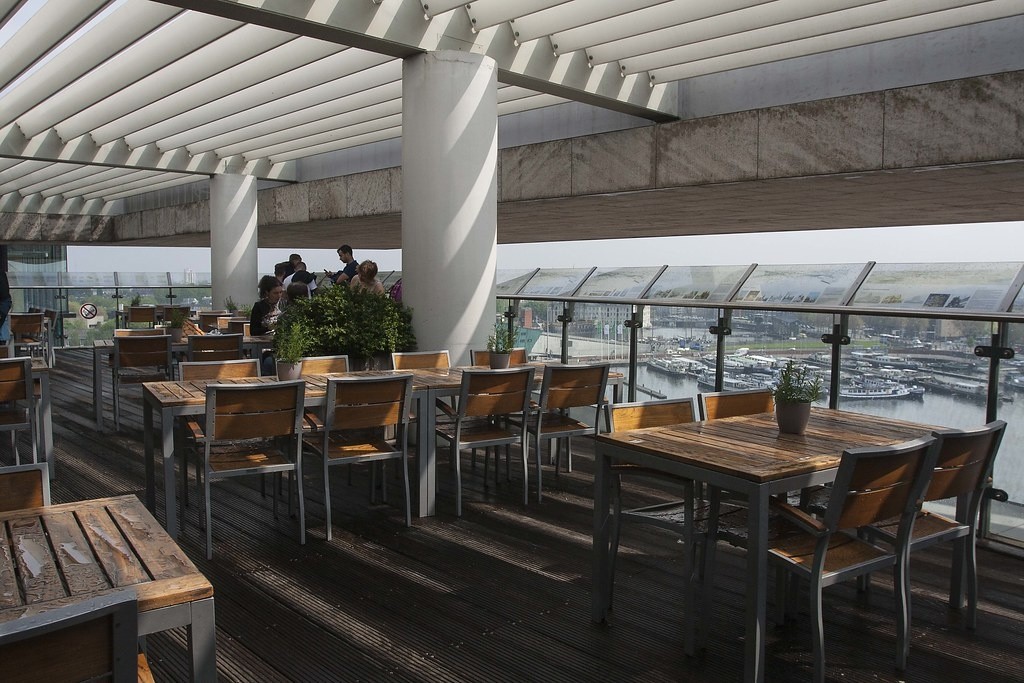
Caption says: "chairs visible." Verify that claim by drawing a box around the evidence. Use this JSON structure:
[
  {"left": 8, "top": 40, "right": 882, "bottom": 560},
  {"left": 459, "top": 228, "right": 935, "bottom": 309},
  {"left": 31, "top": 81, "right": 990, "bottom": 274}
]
[{"left": 0, "top": 298, "right": 1022, "bottom": 683}]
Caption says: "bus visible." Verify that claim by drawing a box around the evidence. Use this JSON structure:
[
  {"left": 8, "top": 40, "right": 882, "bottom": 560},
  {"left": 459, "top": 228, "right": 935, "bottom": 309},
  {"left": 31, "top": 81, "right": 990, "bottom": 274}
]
[{"left": 879, "top": 334, "right": 900, "bottom": 345}]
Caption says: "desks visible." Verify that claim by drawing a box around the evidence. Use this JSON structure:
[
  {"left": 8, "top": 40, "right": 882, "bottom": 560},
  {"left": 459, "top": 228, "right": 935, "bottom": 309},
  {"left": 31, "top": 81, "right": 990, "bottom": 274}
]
[
  {"left": 583, "top": 404, "right": 965, "bottom": 682},
  {"left": 93, "top": 332, "right": 259, "bottom": 429},
  {"left": 115, "top": 308, "right": 197, "bottom": 330},
  {"left": 0, "top": 355, "right": 57, "bottom": 482},
  {"left": 1, "top": 497, "right": 218, "bottom": 682},
  {"left": 0, "top": 317, "right": 54, "bottom": 367},
  {"left": 243, "top": 335, "right": 275, "bottom": 366},
  {"left": 141, "top": 363, "right": 429, "bottom": 544},
  {"left": 379, "top": 362, "right": 625, "bottom": 517}
]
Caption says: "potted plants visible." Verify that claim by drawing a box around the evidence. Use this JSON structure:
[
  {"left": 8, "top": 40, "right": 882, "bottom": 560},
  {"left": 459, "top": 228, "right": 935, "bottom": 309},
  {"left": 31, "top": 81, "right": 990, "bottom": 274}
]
[
  {"left": 486, "top": 314, "right": 520, "bottom": 370},
  {"left": 773, "top": 359, "right": 821, "bottom": 434}
]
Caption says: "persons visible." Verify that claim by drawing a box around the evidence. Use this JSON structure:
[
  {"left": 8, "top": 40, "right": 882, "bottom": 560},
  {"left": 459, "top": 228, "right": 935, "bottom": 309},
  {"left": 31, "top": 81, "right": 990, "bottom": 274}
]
[
  {"left": 274, "top": 254, "right": 328, "bottom": 296},
  {"left": 270, "top": 280, "right": 314, "bottom": 361},
  {"left": 324, "top": 244, "right": 360, "bottom": 290},
  {"left": 350, "top": 259, "right": 384, "bottom": 297},
  {"left": 250, "top": 275, "right": 287, "bottom": 336}
]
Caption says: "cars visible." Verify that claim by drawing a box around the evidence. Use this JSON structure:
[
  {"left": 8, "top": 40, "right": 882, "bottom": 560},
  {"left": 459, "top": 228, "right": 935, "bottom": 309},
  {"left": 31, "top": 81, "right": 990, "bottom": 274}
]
[
  {"left": 912, "top": 339, "right": 921, "bottom": 344},
  {"left": 798, "top": 332, "right": 806, "bottom": 337},
  {"left": 924, "top": 342, "right": 932, "bottom": 347}
]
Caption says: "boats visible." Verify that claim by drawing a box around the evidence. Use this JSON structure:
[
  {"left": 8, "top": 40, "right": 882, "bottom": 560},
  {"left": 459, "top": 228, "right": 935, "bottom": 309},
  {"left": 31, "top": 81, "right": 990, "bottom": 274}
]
[
  {"left": 808, "top": 351, "right": 989, "bottom": 399},
  {"left": 648, "top": 354, "right": 820, "bottom": 396},
  {"left": 999, "top": 357, "right": 1023, "bottom": 402}
]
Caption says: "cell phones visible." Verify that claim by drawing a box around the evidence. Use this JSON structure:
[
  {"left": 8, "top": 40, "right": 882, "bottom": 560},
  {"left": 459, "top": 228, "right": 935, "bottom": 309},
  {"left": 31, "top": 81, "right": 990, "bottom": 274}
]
[{"left": 324, "top": 269, "right": 329, "bottom": 274}]
